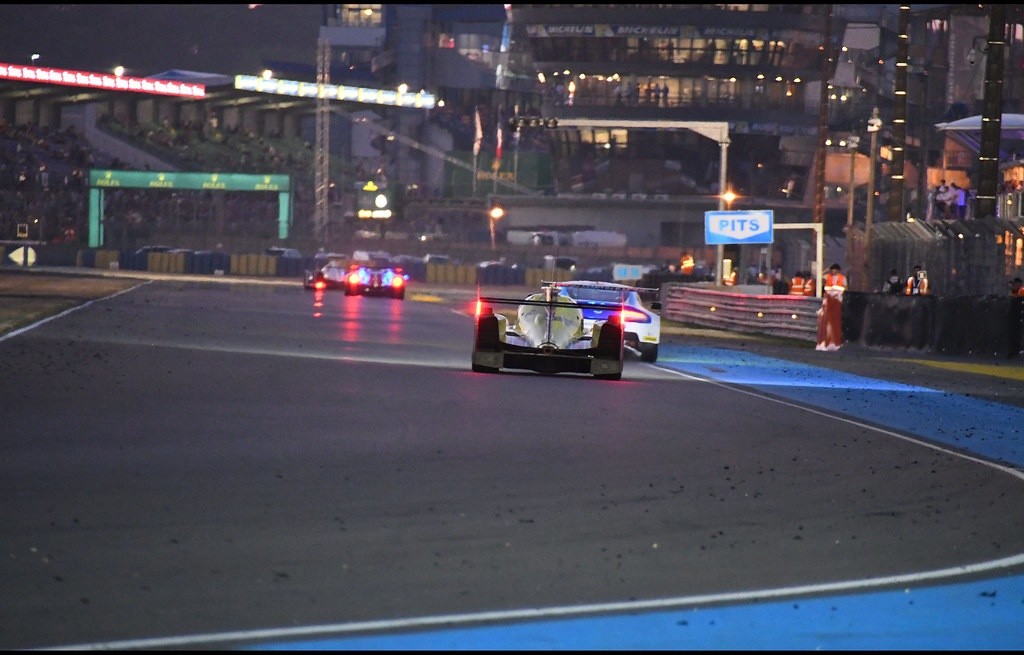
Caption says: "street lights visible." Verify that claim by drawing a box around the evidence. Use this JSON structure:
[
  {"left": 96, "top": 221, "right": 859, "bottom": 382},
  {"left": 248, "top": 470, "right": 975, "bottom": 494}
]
[{"left": 490, "top": 208, "right": 503, "bottom": 248}]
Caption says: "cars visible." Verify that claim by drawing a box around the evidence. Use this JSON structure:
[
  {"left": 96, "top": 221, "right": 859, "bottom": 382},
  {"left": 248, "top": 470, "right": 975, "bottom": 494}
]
[
  {"left": 344, "top": 265, "right": 409, "bottom": 299},
  {"left": 556, "top": 280, "right": 662, "bottom": 362},
  {"left": 304, "top": 261, "right": 344, "bottom": 291}
]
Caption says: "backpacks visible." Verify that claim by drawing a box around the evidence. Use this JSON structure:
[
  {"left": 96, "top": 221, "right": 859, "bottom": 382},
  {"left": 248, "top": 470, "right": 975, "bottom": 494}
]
[{"left": 887, "top": 278, "right": 903, "bottom": 293}]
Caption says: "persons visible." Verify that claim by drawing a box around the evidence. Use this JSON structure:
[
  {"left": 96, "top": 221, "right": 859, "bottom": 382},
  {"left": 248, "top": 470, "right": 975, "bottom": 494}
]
[
  {"left": 614, "top": 79, "right": 670, "bottom": 109},
  {"left": 936, "top": 176, "right": 968, "bottom": 219},
  {"left": 678, "top": 250, "right": 695, "bottom": 277},
  {"left": 1, "top": 111, "right": 443, "bottom": 243},
  {"left": 997, "top": 178, "right": 1024, "bottom": 194},
  {"left": 767, "top": 262, "right": 931, "bottom": 353},
  {"left": 1007, "top": 276, "right": 1024, "bottom": 298}
]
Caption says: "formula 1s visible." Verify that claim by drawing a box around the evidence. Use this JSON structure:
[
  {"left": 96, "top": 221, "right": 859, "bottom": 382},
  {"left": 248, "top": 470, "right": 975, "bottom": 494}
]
[{"left": 472, "top": 284, "right": 624, "bottom": 380}]
